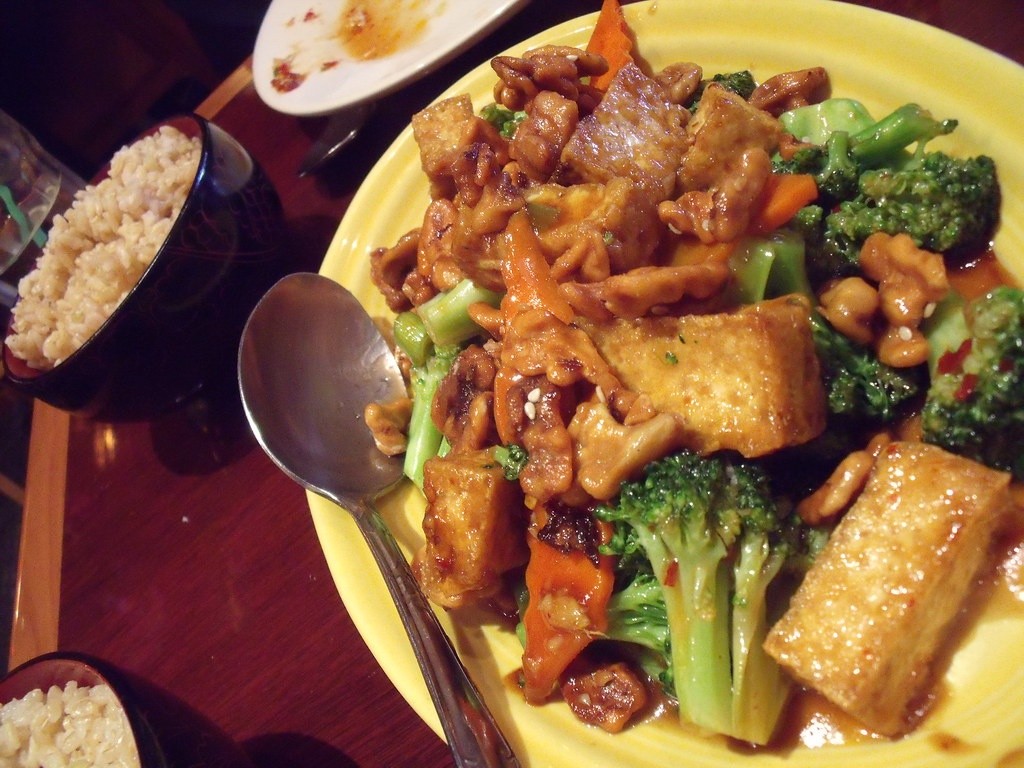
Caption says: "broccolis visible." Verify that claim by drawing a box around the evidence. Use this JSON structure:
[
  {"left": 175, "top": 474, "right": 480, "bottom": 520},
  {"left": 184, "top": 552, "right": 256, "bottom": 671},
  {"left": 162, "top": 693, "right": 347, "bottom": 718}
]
[{"left": 388, "top": 71, "right": 1024, "bottom": 748}]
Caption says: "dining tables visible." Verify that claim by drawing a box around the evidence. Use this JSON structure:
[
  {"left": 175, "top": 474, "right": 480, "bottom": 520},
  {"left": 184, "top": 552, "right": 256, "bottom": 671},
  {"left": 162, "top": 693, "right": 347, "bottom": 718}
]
[{"left": 1, "top": 3, "right": 1024, "bottom": 767}]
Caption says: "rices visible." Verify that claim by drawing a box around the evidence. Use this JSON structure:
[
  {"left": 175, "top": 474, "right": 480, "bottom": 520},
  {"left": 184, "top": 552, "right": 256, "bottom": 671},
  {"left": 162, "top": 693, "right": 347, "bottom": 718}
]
[
  {"left": 0, "top": 680, "right": 140, "bottom": 768},
  {"left": 3, "top": 124, "right": 202, "bottom": 369}
]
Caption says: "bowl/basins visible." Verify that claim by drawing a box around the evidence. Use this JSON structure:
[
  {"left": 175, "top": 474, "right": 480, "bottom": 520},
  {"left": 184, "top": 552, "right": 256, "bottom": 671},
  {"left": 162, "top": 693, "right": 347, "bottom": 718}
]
[
  {"left": 0, "top": 650, "right": 165, "bottom": 768},
  {"left": 3, "top": 113, "right": 294, "bottom": 422}
]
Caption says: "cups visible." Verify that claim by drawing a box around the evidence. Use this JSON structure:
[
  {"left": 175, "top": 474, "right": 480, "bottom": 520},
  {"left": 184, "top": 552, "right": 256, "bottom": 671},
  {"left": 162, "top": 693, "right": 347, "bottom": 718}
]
[{"left": 0, "top": 109, "right": 92, "bottom": 310}]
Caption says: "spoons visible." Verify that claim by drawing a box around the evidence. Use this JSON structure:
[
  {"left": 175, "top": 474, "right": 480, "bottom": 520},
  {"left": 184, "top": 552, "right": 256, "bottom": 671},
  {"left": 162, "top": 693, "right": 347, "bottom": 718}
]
[{"left": 237, "top": 272, "right": 523, "bottom": 768}]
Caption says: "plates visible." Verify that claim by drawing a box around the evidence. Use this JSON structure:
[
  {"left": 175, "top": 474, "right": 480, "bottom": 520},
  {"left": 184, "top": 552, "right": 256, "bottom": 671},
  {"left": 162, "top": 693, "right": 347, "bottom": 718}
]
[
  {"left": 251, "top": 0, "right": 532, "bottom": 117},
  {"left": 292, "top": 0, "right": 1024, "bottom": 768}
]
